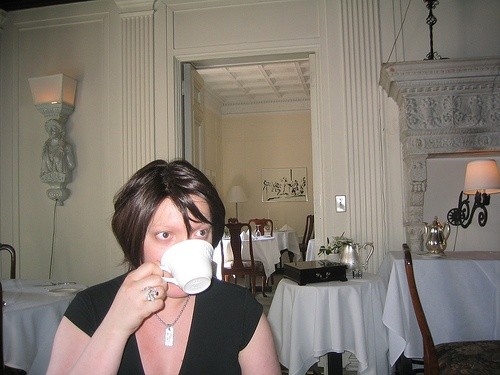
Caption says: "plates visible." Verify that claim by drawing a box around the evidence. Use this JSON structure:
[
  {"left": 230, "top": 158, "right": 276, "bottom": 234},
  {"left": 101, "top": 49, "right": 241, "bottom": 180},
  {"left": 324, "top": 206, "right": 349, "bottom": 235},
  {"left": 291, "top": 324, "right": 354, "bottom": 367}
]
[{"left": 48, "top": 288, "right": 80, "bottom": 294}]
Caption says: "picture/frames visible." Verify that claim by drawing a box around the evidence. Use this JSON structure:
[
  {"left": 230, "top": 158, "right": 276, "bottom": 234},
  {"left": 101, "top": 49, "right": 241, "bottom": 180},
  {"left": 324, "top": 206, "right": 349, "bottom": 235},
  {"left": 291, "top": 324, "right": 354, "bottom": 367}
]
[{"left": 336, "top": 195, "right": 347, "bottom": 213}]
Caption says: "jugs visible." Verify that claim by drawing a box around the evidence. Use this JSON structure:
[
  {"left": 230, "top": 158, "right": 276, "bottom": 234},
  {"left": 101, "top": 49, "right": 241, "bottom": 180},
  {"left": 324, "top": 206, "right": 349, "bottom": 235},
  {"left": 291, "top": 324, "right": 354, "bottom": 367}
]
[{"left": 337, "top": 239, "right": 374, "bottom": 276}]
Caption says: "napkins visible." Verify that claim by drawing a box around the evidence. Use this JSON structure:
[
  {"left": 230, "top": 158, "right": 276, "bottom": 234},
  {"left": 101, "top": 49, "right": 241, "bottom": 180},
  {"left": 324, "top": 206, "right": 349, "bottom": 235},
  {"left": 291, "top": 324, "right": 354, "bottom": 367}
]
[
  {"left": 240, "top": 230, "right": 252, "bottom": 241},
  {"left": 280, "top": 223, "right": 294, "bottom": 231}
]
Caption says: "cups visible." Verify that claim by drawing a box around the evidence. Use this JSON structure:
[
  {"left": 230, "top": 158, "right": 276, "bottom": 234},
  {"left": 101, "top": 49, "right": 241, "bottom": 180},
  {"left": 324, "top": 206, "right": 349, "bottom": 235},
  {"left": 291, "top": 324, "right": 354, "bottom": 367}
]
[{"left": 158, "top": 239, "right": 214, "bottom": 295}]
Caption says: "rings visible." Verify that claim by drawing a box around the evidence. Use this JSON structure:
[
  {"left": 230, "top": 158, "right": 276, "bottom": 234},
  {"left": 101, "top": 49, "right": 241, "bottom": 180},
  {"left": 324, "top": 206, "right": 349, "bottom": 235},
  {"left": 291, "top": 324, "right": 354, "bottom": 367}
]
[{"left": 147, "top": 287, "right": 158, "bottom": 302}]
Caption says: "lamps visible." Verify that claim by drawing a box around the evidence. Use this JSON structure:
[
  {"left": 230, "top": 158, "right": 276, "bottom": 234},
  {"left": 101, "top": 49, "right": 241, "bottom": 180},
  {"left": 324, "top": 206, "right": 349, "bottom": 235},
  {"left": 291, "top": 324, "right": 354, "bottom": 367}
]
[
  {"left": 27, "top": 73, "right": 78, "bottom": 125},
  {"left": 228, "top": 186, "right": 248, "bottom": 223},
  {"left": 447, "top": 160, "right": 500, "bottom": 229}
]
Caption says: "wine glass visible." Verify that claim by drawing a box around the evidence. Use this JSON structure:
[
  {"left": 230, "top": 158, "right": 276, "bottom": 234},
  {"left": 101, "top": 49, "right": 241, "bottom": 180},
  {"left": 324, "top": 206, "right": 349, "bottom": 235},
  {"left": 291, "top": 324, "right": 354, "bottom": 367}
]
[
  {"left": 274, "top": 220, "right": 280, "bottom": 230},
  {"left": 255, "top": 225, "right": 261, "bottom": 238}
]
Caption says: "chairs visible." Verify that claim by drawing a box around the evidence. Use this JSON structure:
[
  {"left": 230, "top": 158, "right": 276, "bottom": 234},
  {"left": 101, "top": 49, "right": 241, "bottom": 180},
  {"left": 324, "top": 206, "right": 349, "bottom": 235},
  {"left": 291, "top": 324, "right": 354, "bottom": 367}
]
[
  {"left": 279, "top": 214, "right": 313, "bottom": 273},
  {"left": 248, "top": 218, "right": 278, "bottom": 285},
  {"left": 402, "top": 243, "right": 500, "bottom": 374},
  {"left": 222, "top": 223, "right": 268, "bottom": 298}
]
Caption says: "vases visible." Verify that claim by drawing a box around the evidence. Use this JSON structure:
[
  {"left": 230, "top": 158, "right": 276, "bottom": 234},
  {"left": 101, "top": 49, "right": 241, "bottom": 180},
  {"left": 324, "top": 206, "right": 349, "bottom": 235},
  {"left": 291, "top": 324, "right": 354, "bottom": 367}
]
[{"left": 327, "top": 253, "right": 340, "bottom": 263}]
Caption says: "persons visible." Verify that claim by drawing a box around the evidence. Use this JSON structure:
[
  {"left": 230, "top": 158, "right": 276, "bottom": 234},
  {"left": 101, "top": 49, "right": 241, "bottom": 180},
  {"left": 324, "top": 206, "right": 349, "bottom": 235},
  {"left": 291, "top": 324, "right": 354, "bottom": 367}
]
[
  {"left": 39, "top": 119, "right": 75, "bottom": 176},
  {"left": 45, "top": 159, "right": 282, "bottom": 375}
]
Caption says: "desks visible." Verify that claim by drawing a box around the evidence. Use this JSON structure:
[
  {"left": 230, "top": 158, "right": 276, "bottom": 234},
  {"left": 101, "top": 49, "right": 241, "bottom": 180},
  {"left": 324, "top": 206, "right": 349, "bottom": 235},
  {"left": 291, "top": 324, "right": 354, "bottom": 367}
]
[
  {"left": 212, "top": 236, "right": 282, "bottom": 287},
  {"left": 266, "top": 271, "right": 397, "bottom": 375},
  {"left": 2, "top": 279, "right": 89, "bottom": 374},
  {"left": 375, "top": 250, "right": 500, "bottom": 374}
]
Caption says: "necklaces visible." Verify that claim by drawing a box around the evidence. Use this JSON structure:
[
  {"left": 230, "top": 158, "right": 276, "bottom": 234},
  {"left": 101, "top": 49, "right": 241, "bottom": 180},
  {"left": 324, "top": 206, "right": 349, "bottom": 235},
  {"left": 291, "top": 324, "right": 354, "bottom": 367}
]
[{"left": 154, "top": 293, "right": 192, "bottom": 347}]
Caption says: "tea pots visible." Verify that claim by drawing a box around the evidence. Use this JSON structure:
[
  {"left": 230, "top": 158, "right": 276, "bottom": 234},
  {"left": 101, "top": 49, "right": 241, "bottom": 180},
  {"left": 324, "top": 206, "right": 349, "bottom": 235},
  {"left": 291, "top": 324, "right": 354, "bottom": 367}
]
[{"left": 421, "top": 216, "right": 451, "bottom": 256}]
